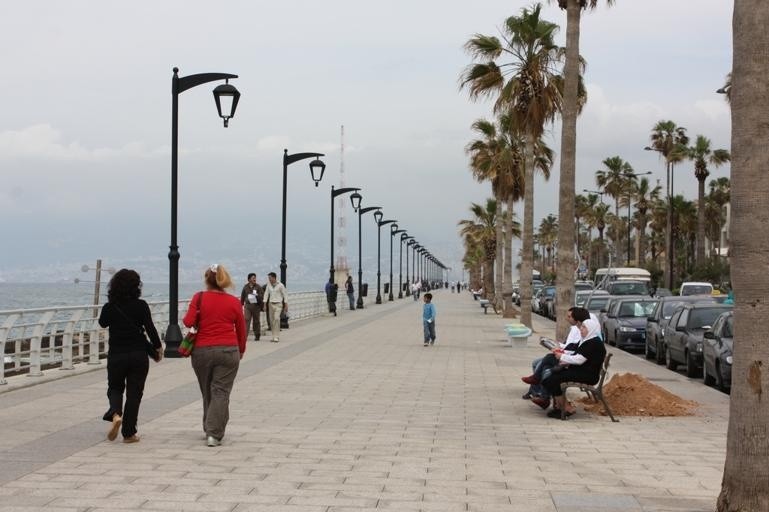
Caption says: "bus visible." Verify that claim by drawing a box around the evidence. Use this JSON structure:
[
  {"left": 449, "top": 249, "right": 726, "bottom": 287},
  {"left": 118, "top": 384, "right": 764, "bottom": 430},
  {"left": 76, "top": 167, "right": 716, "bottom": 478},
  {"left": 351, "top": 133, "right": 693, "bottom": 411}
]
[{"left": 591, "top": 268, "right": 651, "bottom": 290}]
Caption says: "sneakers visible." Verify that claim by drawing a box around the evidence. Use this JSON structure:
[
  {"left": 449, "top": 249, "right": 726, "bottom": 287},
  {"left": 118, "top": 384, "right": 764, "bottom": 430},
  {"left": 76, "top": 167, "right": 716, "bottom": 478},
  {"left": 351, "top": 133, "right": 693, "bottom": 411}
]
[
  {"left": 207, "top": 436, "right": 223, "bottom": 446},
  {"left": 521, "top": 374, "right": 577, "bottom": 418},
  {"left": 108, "top": 415, "right": 140, "bottom": 443}
]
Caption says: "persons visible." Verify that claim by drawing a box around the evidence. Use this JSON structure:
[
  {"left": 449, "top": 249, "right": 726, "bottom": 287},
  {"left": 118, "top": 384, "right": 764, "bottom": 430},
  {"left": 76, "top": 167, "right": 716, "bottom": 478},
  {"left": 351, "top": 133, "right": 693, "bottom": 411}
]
[
  {"left": 98, "top": 269, "right": 162, "bottom": 444},
  {"left": 522, "top": 307, "right": 580, "bottom": 399},
  {"left": 724, "top": 292, "right": 733, "bottom": 304},
  {"left": 520, "top": 308, "right": 591, "bottom": 410},
  {"left": 184, "top": 263, "right": 247, "bottom": 447},
  {"left": 541, "top": 318, "right": 607, "bottom": 419},
  {"left": 242, "top": 271, "right": 491, "bottom": 346}
]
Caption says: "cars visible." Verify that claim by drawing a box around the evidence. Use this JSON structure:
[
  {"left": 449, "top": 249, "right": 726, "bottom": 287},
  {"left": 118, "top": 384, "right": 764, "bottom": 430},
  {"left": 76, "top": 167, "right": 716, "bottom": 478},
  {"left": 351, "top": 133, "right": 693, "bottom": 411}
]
[
  {"left": 663, "top": 305, "right": 733, "bottom": 378},
  {"left": 510, "top": 279, "right": 657, "bottom": 352},
  {"left": 651, "top": 288, "right": 674, "bottom": 298},
  {"left": 701, "top": 310, "right": 733, "bottom": 394}
]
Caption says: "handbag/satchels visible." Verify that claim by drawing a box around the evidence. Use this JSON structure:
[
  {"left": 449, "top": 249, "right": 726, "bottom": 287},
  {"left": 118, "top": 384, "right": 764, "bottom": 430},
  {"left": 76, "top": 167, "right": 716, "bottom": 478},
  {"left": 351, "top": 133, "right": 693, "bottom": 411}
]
[
  {"left": 177, "top": 332, "right": 197, "bottom": 357},
  {"left": 147, "top": 340, "right": 163, "bottom": 362}
]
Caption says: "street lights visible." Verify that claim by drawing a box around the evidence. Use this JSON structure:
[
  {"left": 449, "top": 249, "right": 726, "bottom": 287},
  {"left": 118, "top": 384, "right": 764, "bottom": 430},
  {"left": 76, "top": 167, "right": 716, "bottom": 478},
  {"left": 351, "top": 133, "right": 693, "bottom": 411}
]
[
  {"left": 644, "top": 145, "right": 675, "bottom": 290},
  {"left": 533, "top": 213, "right": 558, "bottom": 278},
  {"left": 596, "top": 169, "right": 652, "bottom": 266},
  {"left": 163, "top": 68, "right": 241, "bottom": 357},
  {"left": 276, "top": 150, "right": 326, "bottom": 327},
  {"left": 388, "top": 228, "right": 446, "bottom": 301},
  {"left": 583, "top": 187, "right": 613, "bottom": 267},
  {"left": 375, "top": 219, "right": 397, "bottom": 305},
  {"left": 356, "top": 203, "right": 383, "bottom": 311},
  {"left": 329, "top": 186, "right": 361, "bottom": 313}
]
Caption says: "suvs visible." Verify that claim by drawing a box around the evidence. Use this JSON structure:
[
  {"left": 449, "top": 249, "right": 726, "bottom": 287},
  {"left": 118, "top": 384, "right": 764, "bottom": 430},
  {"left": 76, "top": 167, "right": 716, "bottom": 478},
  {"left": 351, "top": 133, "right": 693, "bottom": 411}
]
[{"left": 644, "top": 294, "right": 719, "bottom": 365}]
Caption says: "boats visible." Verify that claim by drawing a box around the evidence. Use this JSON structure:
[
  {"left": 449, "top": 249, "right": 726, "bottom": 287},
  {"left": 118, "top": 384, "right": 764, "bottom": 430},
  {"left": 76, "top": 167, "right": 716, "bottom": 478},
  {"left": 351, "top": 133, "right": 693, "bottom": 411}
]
[{"left": 1, "top": 258, "right": 115, "bottom": 377}]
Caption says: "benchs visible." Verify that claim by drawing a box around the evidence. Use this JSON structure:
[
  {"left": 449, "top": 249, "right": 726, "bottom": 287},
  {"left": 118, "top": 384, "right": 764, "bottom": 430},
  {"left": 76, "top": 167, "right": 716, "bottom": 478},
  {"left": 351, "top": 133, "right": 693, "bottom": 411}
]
[
  {"left": 502, "top": 321, "right": 532, "bottom": 349},
  {"left": 481, "top": 296, "right": 501, "bottom": 315},
  {"left": 551, "top": 344, "right": 621, "bottom": 425},
  {"left": 474, "top": 287, "right": 484, "bottom": 301}
]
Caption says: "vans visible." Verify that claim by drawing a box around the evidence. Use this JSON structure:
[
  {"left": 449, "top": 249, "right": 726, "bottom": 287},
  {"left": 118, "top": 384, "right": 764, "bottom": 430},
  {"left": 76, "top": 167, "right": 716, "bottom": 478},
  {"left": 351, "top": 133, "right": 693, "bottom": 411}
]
[{"left": 675, "top": 282, "right": 715, "bottom": 298}]
